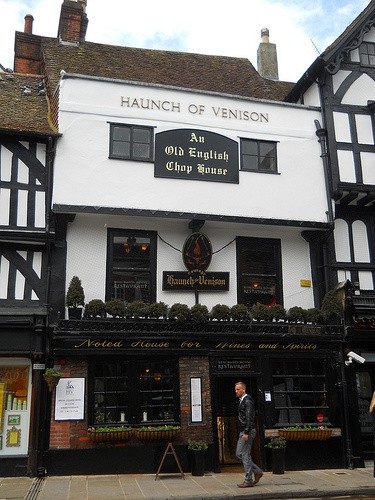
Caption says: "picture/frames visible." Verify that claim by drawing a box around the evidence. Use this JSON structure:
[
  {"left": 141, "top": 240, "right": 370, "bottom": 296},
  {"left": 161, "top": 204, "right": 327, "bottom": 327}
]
[
  {"left": 6, "top": 427, "right": 21, "bottom": 447},
  {"left": 8, "top": 415, "right": 20, "bottom": 425},
  {"left": 0, "top": 436, "right": 2, "bottom": 449}
]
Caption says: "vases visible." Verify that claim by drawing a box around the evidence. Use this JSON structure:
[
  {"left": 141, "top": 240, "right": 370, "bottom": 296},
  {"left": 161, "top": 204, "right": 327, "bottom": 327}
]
[
  {"left": 136, "top": 429, "right": 181, "bottom": 439},
  {"left": 280, "top": 428, "right": 333, "bottom": 439},
  {"left": 90, "top": 430, "right": 132, "bottom": 441}
]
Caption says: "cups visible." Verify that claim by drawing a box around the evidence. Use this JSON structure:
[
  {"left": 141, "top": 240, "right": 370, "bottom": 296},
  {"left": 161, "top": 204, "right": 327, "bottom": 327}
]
[{"left": 7, "top": 394, "right": 26, "bottom": 410}]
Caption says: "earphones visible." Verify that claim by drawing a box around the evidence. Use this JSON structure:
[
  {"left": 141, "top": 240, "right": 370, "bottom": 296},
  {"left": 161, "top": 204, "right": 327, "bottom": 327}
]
[{"left": 242, "top": 389, "right": 244, "bottom": 392}]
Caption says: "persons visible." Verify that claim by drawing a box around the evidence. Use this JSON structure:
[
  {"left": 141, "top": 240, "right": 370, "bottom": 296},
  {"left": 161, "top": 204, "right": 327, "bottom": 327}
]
[{"left": 234, "top": 382, "right": 263, "bottom": 487}]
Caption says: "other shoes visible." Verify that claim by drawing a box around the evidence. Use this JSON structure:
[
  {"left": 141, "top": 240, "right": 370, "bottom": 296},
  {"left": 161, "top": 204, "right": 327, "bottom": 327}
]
[
  {"left": 253, "top": 472, "right": 263, "bottom": 484},
  {"left": 239, "top": 482, "right": 254, "bottom": 488}
]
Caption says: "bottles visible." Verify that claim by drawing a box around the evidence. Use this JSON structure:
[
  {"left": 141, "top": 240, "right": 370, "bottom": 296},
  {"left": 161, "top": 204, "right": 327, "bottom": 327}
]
[
  {"left": 121, "top": 411, "right": 125, "bottom": 421},
  {"left": 143, "top": 411, "right": 147, "bottom": 421}
]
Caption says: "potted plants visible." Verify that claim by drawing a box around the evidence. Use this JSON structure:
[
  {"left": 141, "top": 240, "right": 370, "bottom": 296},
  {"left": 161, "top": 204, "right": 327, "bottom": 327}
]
[
  {"left": 269, "top": 436, "right": 287, "bottom": 473},
  {"left": 44, "top": 367, "right": 62, "bottom": 392},
  {"left": 321, "top": 289, "right": 344, "bottom": 325},
  {"left": 65, "top": 277, "right": 84, "bottom": 321},
  {"left": 189, "top": 438, "right": 209, "bottom": 475}
]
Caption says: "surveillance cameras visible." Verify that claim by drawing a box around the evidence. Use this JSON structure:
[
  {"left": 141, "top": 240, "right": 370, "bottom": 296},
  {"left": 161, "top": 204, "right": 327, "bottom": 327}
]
[{"left": 345, "top": 351, "right": 366, "bottom": 365}]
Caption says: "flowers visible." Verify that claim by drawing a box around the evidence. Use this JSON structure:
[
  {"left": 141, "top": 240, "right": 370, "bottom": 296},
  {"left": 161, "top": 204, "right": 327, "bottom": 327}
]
[
  {"left": 136, "top": 424, "right": 179, "bottom": 432},
  {"left": 88, "top": 425, "right": 132, "bottom": 433},
  {"left": 282, "top": 424, "right": 330, "bottom": 430}
]
